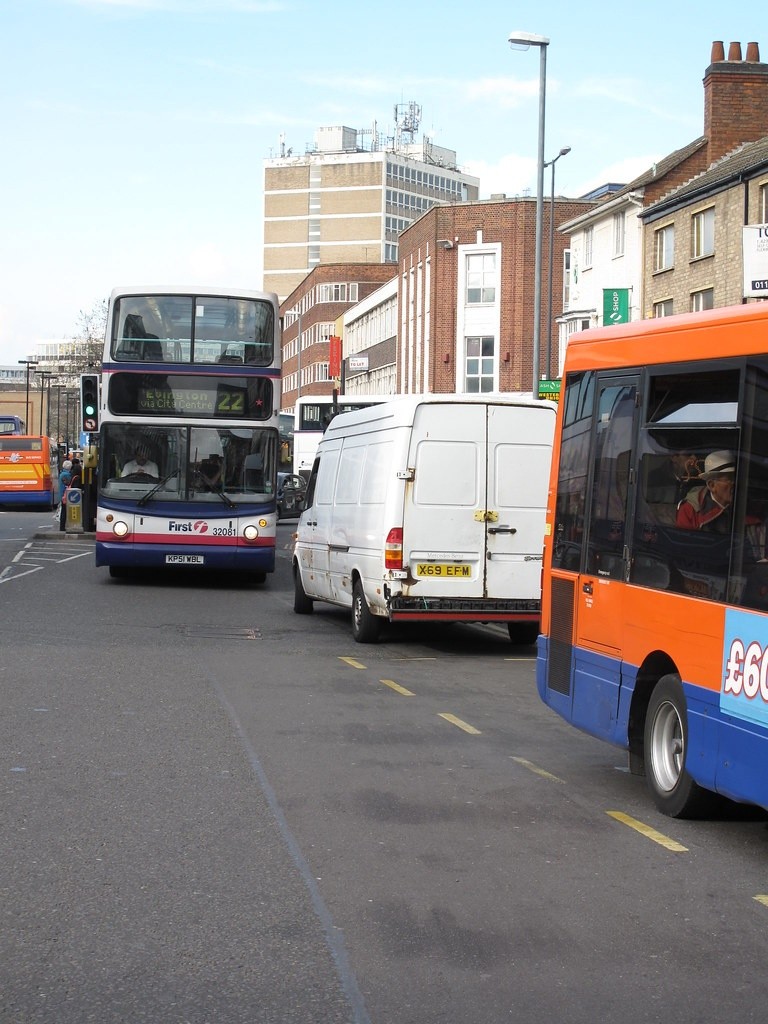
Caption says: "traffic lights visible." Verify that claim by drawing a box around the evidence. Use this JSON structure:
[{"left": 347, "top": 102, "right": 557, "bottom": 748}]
[{"left": 80, "top": 374, "right": 101, "bottom": 433}]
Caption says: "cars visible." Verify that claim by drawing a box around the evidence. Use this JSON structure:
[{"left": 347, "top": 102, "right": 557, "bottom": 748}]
[{"left": 276, "top": 471, "right": 307, "bottom": 521}]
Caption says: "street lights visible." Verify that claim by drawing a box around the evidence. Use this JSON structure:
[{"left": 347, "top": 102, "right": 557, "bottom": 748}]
[
  {"left": 60, "top": 391, "right": 74, "bottom": 445},
  {"left": 41, "top": 375, "right": 57, "bottom": 438},
  {"left": 18, "top": 360, "right": 39, "bottom": 436},
  {"left": 32, "top": 370, "right": 52, "bottom": 437},
  {"left": 51, "top": 384, "right": 66, "bottom": 441},
  {"left": 506, "top": 29, "right": 588, "bottom": 400},
  {"left": 285, "top": 310, "right": 303, "bottom": 396},
  {"left": 70, "top": 396, "right": 83, "bottom": 451}
]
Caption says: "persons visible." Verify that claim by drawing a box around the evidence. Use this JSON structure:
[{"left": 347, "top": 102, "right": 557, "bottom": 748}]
[
  {"left": 119, "top": 445, "right": 162, "bottom": 480},
  {"left": 675, "top": 450, "right": 763, "bottom": 533},
  {"left": 58, "top": 460, "right": 78, "bottom": 531},
  {"left": 70, "top": 458, "right": 82, "bottom": 485},
  {"left": 647, "top": 435, "right": 707, "bottom": 506}
]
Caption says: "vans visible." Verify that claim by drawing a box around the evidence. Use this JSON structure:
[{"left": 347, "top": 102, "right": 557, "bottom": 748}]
[{"left": 291, "top": 392, "right": 558, "bottom": 653}]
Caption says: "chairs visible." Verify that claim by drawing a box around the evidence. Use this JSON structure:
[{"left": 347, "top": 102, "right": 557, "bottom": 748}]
[
  {"left": 116, "top": 344, "right": 272, "bottom": 369},
  {"left": 596, "top": 451, "right": 678, "bottom": 532}
]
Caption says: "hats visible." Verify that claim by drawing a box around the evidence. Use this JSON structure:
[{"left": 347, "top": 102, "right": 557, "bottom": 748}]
[{"left": 696, "top": 449, "right": 740, "bottom": 480}]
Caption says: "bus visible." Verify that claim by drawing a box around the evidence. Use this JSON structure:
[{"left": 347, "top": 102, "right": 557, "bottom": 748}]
[
  {"left": 0, "top": 415, "right": 27, "bottom": 436},
  {"left": 278, "top": 412, "right": 296, "bottom": 472},
  {"left": 93, "top": 284, "right": 281, "bottom": 587},
  {"left": 537, "top": 301, "right": 767, "bottom": 817},
  {"left": 0, "top": 435, "right": 65, "bottom": 511},
  {"left": 293, "top": 394, "right": 401, "bottom": 514}
]
